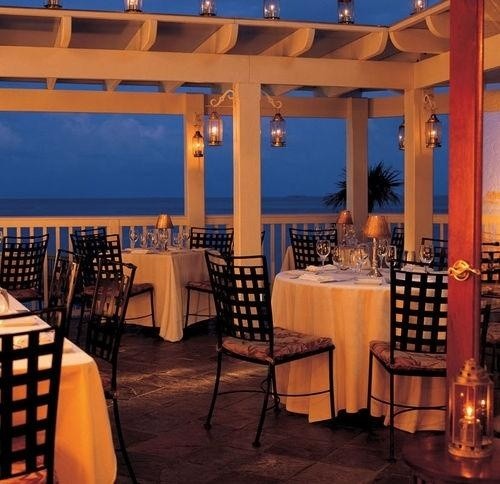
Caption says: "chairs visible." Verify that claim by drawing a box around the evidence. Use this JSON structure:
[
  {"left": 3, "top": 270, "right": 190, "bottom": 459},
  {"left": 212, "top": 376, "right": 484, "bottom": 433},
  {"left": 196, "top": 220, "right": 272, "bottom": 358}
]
[
  {"left": 50, "top": 250, "right": 74, "bottom": 332},
  {"left": 182, "top": 229, "right": 234, "bottom": 250},
  {"left": 419, "top": 236, "right": 500, "bottom": 285},
  {"left": 1, "top": 234, "right": 49, "bottom": 300},
  {"left": 181, "top": 250, "right": 236, "bottom": 340},
  {"left": 71, "top": 234, "right": 159, "bottom": 339},
  {"left": 80, "top": 258, "right": 147, "bottom": 474},
  {"left": 365, "top": 258, "right": 454, "bottom": 459},
  {"left": 288, "top": 229, "right": 350, "bottom": 267},
  {"left": 2, "top": 323, "right": 72, "bottom": 482},
  {"left": 193, "top": 248, "right": 340, "bottom": 441}
]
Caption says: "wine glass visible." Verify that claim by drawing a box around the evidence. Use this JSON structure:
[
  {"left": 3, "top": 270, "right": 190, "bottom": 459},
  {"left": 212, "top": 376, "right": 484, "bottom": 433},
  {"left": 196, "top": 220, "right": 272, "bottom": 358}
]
[
  {"left": 315, "top": 237, "right": 436, "bottom": 281},
  {"left": 128, "top": 228, "right": 191, "bottom": 250}
]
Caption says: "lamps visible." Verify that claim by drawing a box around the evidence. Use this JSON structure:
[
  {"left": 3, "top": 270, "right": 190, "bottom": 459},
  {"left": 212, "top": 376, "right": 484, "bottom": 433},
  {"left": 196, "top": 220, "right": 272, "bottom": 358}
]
[
  {"left": 425, "top": 114, "right": 442, "bottom": 148},
  {"left": 124, "top": 0, "right": 144, "bottom": 12},
  {"left": 336, "top": 208, "right": 354, "bottom": 246},
  {"left": 192, "top": 113, "right": 204, "bottom": 157},
  {"left": 208, "top": 108, "right": 224, "bottom": 146},
  {"left": 410, "top": 0, "right": 427, "bottom": 15},
  {"left": 43, "top": 0, "right": 62, "bottom": 8},
  {"left": 362, "top": 216, "right": 391, "bottom": 276},
  {"left": 270, "top": 109, "right": 286, "bottom": 147},
  {"left": 156, "top": 214, "right": 174, "bottom": 250},
  {"left": 200, "top": 0, "right": 216, "bottom": 16},
  {"left": 337, "top": 0, "right": 354, "bottom": 24},
  {"left": 397, "top": 122, "right": 405, "bottom": 150},
  {"left": 263, "top": 0, "right": 281, "bottom": 19},
  {"left": 449, "top": 357, "right": 495, "bottom": 460}
]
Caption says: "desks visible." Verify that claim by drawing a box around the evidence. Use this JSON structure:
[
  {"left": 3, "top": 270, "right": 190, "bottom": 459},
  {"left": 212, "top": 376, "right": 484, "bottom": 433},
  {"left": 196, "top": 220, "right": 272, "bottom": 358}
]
[
  {"left": 399, "top": 433, "right": 500, "bottom": 482},
  {"left": 268, "top": 266, "right": 455, "bottom": 435},
  {"left": 0, "top": 287, "right": 121, "bottom": 483},
  {"left": 101, "top": 241, "right": 229, "bottom": 345}
]
